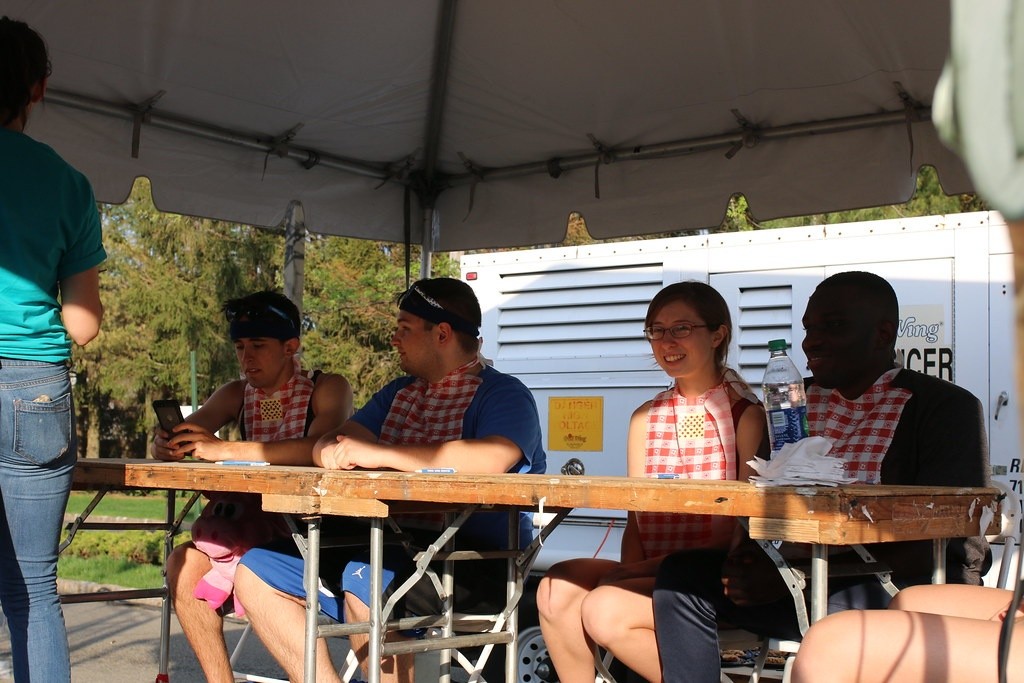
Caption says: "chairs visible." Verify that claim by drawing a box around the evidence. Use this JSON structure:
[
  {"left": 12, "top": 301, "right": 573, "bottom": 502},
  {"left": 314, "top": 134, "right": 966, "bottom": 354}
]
[{"left": 594, "top": 478, "right": 1023, "bottom": 683}]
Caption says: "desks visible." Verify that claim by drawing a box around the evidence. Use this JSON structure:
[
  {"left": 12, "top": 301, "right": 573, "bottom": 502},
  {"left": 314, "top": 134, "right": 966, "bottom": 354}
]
[{"left": 56, "top": 459, "right": 1006, "bottom": 683}]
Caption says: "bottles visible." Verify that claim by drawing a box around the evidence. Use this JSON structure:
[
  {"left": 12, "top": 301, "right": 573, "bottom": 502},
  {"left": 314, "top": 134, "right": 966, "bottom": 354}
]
[{"left": 764, "top": 338, "right": 810, "bottom": 459}]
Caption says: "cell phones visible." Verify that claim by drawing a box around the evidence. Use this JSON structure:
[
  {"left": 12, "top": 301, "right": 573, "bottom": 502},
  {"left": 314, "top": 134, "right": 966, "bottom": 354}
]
[{"left": 152, "top": 399, "right": 195, "bottom": 456}]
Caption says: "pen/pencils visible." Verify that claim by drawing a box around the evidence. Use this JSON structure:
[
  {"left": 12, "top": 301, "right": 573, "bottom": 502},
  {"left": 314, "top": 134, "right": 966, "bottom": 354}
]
[
  {"left": 415, "top": 468, "right": 458, "bottom": 474},
  {"left": 648, "top": 473, "right": 690, "bottom": 479},
  {"left": 215, "top": 460, "right": 271, "bottom": 466}
]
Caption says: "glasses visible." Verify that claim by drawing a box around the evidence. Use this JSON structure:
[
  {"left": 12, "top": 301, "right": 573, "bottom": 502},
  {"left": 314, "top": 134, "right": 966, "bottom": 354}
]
[
  {"left": 226, "top": 300, "right": 295, "bottom": 335},
  {"left": 408, "top": 281, "right": 444, "bottom": 316},
  {"left": 643, "top": 324, "right": 712, "bottom": 341}
]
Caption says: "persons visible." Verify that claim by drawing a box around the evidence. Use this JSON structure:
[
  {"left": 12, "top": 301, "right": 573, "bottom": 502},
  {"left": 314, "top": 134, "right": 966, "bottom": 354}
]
[
  {"left": 649, "top": 269, "right": 992, "bottom": 683},
  {"left": 535, "top": 281, "right": 771, "bottom": 683},
  {"left": 233, "top": 276, "right": 549, "bottom": 683},
  {"left": 789, "top": 583, "right": 1024, "bottom": 683},
  {"left": 0, "top": 14, "right": 114, "bottom": 682},
  {"left": 150, "top": 290, "right": 354, "bottom": 683}
]
